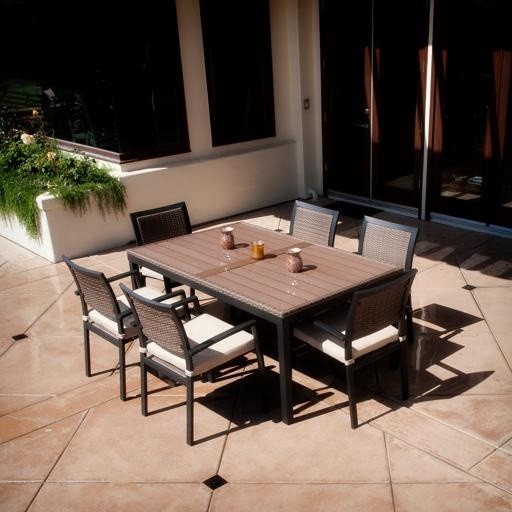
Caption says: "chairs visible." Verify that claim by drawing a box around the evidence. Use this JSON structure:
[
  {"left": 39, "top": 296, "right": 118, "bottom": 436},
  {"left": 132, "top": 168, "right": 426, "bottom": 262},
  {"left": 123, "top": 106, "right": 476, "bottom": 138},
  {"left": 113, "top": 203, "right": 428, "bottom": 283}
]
[
  {"left": 284, "top": 199, "right": 341, "bottom": 252},
  {"left": 61, "top": 256, "right": 194, "bottom": 404},
  {"left": 117, "top": 286, "right": 280, "bottom": 445},
  {"left": 292, "top": 268, "right": 418, "bottom": 428},
  {"left": 131, "top": 200, "right": 198, "bottom": 302},
  {"left": 356, "top": 214, "right": 418, "bottom": 275}
]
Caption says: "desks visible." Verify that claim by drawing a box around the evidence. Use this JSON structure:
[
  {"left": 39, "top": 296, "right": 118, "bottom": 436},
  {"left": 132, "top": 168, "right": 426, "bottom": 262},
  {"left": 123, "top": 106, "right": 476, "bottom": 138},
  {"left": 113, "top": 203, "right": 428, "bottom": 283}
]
[{"left": 124, "top": 219, "right": 416, "bottom": 425}]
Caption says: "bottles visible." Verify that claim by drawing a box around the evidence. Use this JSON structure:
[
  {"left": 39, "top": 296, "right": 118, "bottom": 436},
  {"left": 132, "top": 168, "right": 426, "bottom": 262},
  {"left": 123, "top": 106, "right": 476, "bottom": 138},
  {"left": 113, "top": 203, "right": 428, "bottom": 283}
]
[
  {"left": 287, "top": 247, "right": 302, "bottom": 273},
  {"left": 220, "top": 227, "right": 235, "bottom": 249}
]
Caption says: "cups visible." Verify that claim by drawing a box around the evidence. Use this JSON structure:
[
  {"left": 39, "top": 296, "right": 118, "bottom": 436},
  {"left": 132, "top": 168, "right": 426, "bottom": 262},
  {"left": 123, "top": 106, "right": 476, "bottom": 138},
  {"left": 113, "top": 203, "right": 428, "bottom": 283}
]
[{"left": 251, "top": 241, "right": 264, "bottom": 261}]
